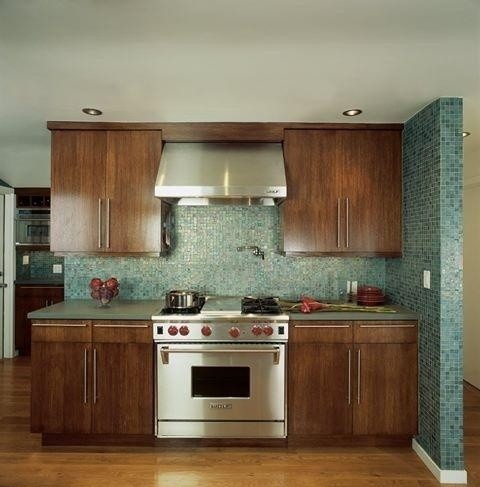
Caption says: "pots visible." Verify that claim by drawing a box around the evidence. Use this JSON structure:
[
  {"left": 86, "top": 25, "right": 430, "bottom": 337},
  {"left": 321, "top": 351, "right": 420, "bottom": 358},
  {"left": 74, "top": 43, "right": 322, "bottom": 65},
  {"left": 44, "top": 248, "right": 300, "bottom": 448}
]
[{"left": 165, "top": 289, "right": 201, "bottom": 308}]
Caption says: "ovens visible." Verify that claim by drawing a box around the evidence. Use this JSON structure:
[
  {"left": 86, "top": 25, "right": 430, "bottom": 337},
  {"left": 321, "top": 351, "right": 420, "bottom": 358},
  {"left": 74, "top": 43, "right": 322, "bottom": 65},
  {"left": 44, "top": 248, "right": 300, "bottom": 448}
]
[{"left": 153, "top": 322, "right": 289, "bottom": 438}]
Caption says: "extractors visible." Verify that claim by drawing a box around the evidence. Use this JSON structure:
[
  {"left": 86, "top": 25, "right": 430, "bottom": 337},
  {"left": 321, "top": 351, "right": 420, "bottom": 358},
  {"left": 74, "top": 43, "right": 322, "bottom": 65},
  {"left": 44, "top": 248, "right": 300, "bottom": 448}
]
[{"left": 155, "top": 142, "right": 287, "bottom": 210}]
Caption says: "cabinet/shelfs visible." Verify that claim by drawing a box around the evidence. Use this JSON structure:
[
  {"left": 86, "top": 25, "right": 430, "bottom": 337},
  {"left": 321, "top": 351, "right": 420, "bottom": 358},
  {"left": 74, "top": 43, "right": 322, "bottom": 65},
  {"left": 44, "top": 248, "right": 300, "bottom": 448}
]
[
  {"left": 47, "top": 121, "right": 167, "bottom": 257},
  {"left": 288, "top": 319, "right": 418, "bottom": 446},
  {"left": 14, "top": 284, "right": 64, "bottom": 356},
  {"left": 14, "top": 188, "right": 50, "bottom": 208},
  {"left": 30, "top": 319, "right": 154, "bottom": 447},
  {"left": 281, "top": 122, "right": 404, "bottom": 258}
]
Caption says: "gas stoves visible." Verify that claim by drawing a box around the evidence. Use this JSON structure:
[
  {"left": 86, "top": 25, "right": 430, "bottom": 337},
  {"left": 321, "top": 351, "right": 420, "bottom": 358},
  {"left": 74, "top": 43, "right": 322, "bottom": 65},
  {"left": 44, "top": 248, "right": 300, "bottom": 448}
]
[{"left": 151, "top": 293, "right": 289, "bottom": 322}]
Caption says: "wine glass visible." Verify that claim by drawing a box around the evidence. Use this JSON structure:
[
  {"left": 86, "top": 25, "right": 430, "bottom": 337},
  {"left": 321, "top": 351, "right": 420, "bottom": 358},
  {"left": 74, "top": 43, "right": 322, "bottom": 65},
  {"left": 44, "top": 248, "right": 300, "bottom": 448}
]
[{"left": 89, "top": 286, "right": 118, "bottom": 308}]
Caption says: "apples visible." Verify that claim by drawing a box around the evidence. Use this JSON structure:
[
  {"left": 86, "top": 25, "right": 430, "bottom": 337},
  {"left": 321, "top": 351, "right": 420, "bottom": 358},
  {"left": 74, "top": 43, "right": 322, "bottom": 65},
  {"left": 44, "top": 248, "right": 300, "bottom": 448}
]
[{"left": 89, "top": 276, "right": 119, "bottom": 300}]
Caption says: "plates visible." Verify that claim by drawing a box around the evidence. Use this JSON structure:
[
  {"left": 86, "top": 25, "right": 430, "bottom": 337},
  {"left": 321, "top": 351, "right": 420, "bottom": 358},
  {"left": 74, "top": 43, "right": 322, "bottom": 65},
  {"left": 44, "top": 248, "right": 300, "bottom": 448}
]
[{"left": 347, "top": 285, "right": 391, "bottom": 306}]
